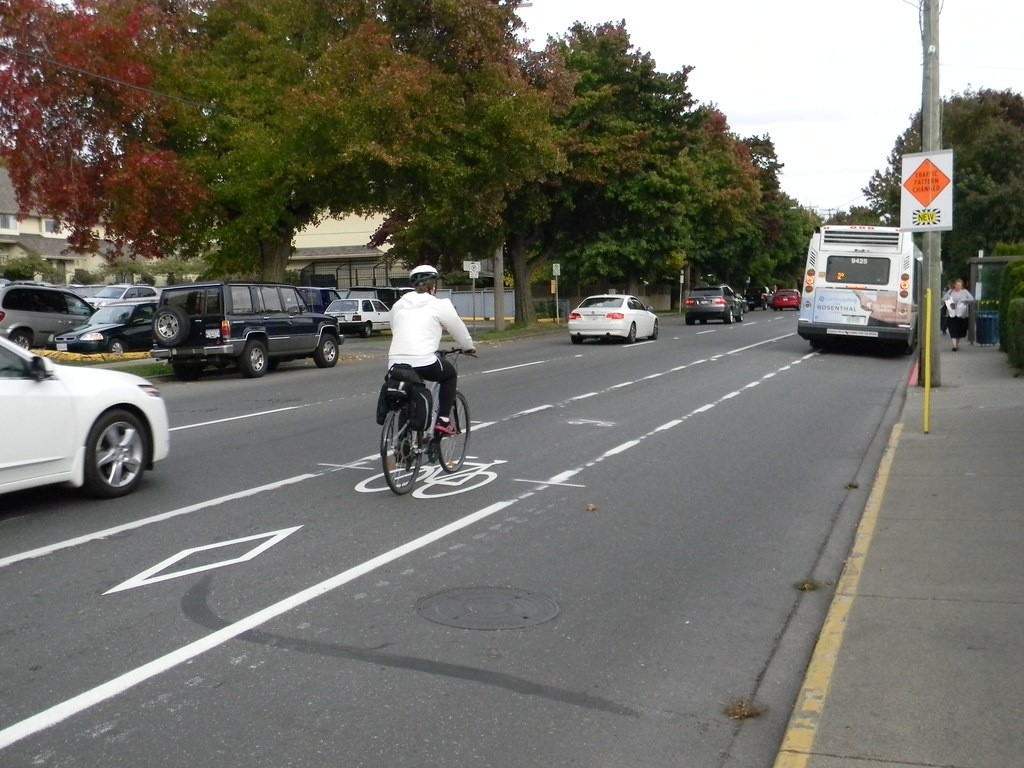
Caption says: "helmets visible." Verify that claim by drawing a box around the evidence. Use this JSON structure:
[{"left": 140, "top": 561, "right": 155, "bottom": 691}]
[{"left": 409, "top": 264, "right": 439, "bottom": 287}]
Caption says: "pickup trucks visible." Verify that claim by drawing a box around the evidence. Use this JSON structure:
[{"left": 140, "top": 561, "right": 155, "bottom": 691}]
[{"left": 745, "top": 286, "right": 773, "bottom": 310}]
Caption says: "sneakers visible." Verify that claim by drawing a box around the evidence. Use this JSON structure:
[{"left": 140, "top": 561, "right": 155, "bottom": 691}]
[{"left": 434, "top": 418, "right": 457, "bottom": 434}]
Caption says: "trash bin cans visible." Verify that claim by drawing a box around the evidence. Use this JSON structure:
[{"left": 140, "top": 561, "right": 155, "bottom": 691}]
[{"left": 976, "top": 315, "right": 1000, "bottom": 347}]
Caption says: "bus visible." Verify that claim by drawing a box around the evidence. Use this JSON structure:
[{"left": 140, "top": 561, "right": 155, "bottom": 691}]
[{"left": 796, "top": 224, "right": 924, "bottom": 353}]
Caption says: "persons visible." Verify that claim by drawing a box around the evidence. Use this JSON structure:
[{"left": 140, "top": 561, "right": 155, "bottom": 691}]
[
  {"left": 388, "top": 265, "right": 476, "bottom": 461},
  {"left": 760, "top": 284, "right": 776, "bottom": 310},
  {"left": 942, "top": 279, "right": 974, "bottom": 352}
]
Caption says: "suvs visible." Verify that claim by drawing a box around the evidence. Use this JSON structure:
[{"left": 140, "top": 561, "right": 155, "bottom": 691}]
[
  {"left": 685, "top": 283, "right": 747, "bottom": 326},
  {"left": 152, "top": 280, "right": 345, "bottom": 379}
]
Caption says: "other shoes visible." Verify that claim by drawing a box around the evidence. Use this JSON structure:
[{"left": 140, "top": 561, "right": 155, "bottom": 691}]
[{"left": 952, "top": 347, "right": 959, "bottom": 351}]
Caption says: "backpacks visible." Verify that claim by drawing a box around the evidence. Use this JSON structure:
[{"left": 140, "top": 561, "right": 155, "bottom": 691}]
[{"left": 376, "top": 363, "right": 433, "bottom": 432}]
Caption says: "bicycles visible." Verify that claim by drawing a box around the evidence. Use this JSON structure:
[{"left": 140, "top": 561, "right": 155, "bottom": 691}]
[{"left": 379, "top": 348, "right": 474, "bottom": 495}]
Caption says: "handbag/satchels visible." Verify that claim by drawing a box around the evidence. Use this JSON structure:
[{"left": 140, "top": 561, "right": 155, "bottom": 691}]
[{"left": 940, "top": 298, "right": 967, "bottom": 331}]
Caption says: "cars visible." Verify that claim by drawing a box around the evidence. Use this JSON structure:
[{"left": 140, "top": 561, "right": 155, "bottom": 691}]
[
  {"left": 567, "top": 293, "right": 661, "bottom": 347},
  {"left": 773, "top": 286, "right": 800, "bottom": 312},
  {"left": 1, "top": 333, "right": 171, "bottom": 498},
  {"left": 0, "top": 278, "right": 164, "bottom": 352},
  {"left": 323, "top": 297, "right": 393, "bottom": 338},
  {"left": 296, "top": 284, "right": 341, "bottom": 313}
]
